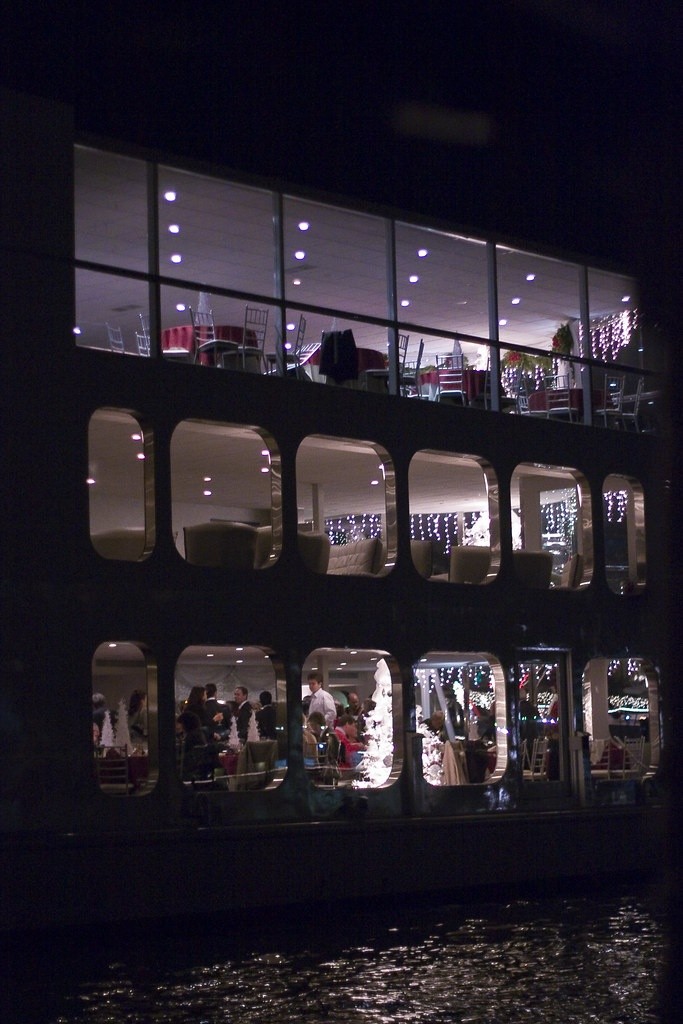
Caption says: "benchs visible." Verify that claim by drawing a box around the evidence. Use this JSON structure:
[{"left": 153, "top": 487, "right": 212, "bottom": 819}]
[{"left": 89, "top": 520, "right": 645, "bottom": 589}]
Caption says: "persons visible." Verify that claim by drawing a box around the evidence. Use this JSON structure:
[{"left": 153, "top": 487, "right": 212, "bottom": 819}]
[
  {"left": 234, "top": 687, "right": 253, "bottom": 749},
  {"left": 126, "top": 688, "right": 148, "bottom": 748},
  {"left": 462, "top": 702, "right": 496, "bottom": 783},
  {"left": 307, "top": 670, "right": 338, "bottom": 729},
  {"left": 92, "top": 692, "right": 118, "bottom": 738},
  {"left": 445, "top": 693, "right": 464, "bottom": 737},
  {"left": 417, "top": 711, "right": 450, "bottom": 744},
  {"left": 307, "top": 711, "right": 338, "bottom": 782},
  {"left": 335, "top": 715, "right": 365, "bottom": 778},
  {"left": 177, "top": 683, "right": 238, "bottom": 776},
  {"left": 255, "top": 691, "right": 277, "bottom": 740},
  {"left": 346, "top": 691, "right": 376, "bottom": 734}
]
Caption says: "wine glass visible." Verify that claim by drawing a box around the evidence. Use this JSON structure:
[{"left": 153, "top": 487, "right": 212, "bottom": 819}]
[{"left": 217, "top": 712, "right": 223, "bottom": 726}]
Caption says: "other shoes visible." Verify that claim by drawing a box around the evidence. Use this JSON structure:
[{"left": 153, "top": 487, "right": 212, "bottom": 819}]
[{"left": 127, "top": 781, "right": 146, "bottom": 795}]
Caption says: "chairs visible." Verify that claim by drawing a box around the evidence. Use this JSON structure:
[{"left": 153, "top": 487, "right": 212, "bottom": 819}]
[
  {"left": 104, "top": 303, "right": 646, "bottom": 436},
  {"left": 94, "top": 738, "right": 658, "bottom": 798}
]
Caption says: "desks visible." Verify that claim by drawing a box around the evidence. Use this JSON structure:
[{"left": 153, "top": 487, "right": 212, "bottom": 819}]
[
  {"left": 99, "top": 752, "right": 239, "bottom": 784},
  {"left": 408, "top": 369, "right": 507, "bottom": 414},
  {"left": 527, "top": 388, "right": 619, "bottom": 431},
  {"left": 546, "top": 747, "right": 629, "bottom": 777},
  {"left": 160, "top": 325, "right": 262, "bottom": 375},
  {"left": 295, "top": 347, "right": 389, "bottom": 395}
]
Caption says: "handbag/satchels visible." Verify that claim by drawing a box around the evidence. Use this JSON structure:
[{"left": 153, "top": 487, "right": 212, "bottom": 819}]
[{"left": 132, "top": 719, "right": 144, "bottom": 735}]
